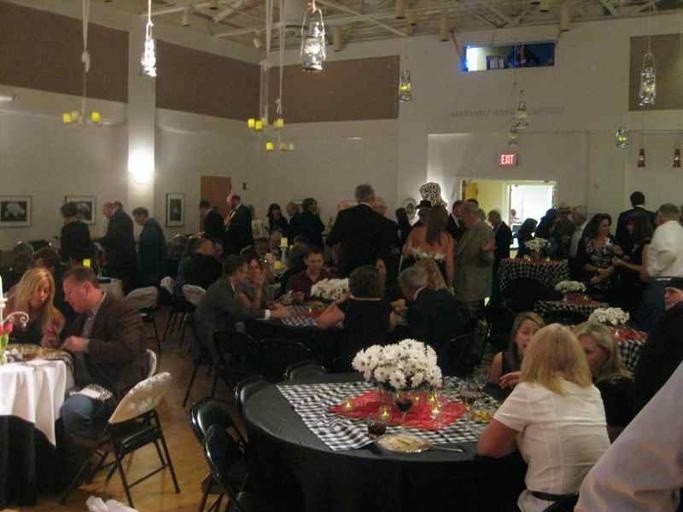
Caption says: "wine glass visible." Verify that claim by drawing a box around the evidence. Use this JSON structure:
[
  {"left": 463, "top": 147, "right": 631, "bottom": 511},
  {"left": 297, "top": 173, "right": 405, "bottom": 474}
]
[{"left": 367, "top": 367, "right": 495, "bottom": 437}]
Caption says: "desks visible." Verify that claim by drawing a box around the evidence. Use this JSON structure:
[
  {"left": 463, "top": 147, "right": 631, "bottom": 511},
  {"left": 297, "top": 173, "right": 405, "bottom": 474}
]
[
  {"left": 89, "top": 276, "right": 123, "bottom": 301},
  {"left": 1, "top": 343, "right": 76, "bottom": 508}
]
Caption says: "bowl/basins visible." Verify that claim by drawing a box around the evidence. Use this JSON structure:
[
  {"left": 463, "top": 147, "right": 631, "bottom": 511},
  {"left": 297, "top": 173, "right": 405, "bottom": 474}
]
[{"left": 6, "top": 342, "right": 39, "bottom": 362}]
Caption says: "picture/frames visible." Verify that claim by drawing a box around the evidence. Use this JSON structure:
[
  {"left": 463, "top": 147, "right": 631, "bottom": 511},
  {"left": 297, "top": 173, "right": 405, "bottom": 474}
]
[
  {"left": 59, "top": 195, "right": 96, "bottom": 226},
  {"left": 0, "top": 192, "right": 34, "bottom": 230},
  {"left": 164, "top": 189, "right": 185, "bottom": 228}
]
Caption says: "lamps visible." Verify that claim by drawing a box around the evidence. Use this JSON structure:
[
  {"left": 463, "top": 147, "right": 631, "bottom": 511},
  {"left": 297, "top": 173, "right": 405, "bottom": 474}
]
[
  {"left": 672, "top": 8, "right": 683, "bottom": 170},
  {"left": 636, "top": 4, "right": 647, "bottom": 169},
  {"left": 247, "top": 3, "right": 296, "bottom": 153},
  {"left": 614, "top": 16, "right": 631, "bottom": 153},
  {"left": 503, "top": 32, "right": 531, "bottom": 152},
  {"left": 636, "top": 6, "right": 661, "bottom": 107},
  {"left": 61, "top": 0, "right": 108, "bottom": 127},
  {"left": 138, "top": 0, "right": 161, "bottom": 79},
  {"left": 395, "top": 34, "right": 417, "bottom": 104},
  {"left": 299, "top": 2, "right": 329, "bottom": 74},
  {"left": 181, "top": 2, "right": 572, "bottom": 52}
]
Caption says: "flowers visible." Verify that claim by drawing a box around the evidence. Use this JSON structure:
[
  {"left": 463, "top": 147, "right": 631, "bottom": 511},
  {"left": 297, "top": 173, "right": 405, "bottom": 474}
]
[
  {"left": 352, "top": 337, "right": 443, "bottom": 394},
  {"left": 555, "top": 280, "right": 587, "bottom": 294},
  {"left": 310, "top": 277, "right": 350, "bottom": 302},
  {"left": 588, "top": 306, "right": 631, "bottom": 327}
]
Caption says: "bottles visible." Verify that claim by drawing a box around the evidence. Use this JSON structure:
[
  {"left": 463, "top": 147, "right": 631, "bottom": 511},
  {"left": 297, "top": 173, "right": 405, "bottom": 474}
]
[{"left": 514, "top": 249, "right": 534, "bottom": 265}]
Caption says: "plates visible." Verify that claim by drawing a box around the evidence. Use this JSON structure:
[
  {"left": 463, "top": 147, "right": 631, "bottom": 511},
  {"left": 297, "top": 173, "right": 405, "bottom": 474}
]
[{"left": 373, "top": 431, "right": 433, "bottom": 455}]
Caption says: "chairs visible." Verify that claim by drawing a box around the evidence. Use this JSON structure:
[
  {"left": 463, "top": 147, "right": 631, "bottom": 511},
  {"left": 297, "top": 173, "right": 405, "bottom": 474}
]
[
  {"left": 467, "top": 309, "right": 493, "bottom": 366},
  {"left": 160, "top": 276, "right": 207, "bottom": 351},
  {"left": 56, "top": 347, "right": 180, "bottom": 511},
  {"left": 181, "top": 313, "right": 327, "bottom": 512},
  {"left": 122, "top": 285, "right": 159, "bottom": 346}
]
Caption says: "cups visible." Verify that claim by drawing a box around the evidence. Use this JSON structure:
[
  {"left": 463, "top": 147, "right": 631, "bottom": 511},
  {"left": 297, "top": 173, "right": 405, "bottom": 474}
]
[
  {"left": 343, "top": 396, "right": 352, "bottom": 413},
  {"left": 575, "top": 293, "right": 590, "bottom": 305},
  {"left": 276, "top": 291, "right": 325, "bottom": 317}
]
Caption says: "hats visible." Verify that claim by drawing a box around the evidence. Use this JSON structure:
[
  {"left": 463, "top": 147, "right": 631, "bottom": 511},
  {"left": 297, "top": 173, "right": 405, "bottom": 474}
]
[{"left": 414, "top": 200, "right": 431, "bottom": 210}]
